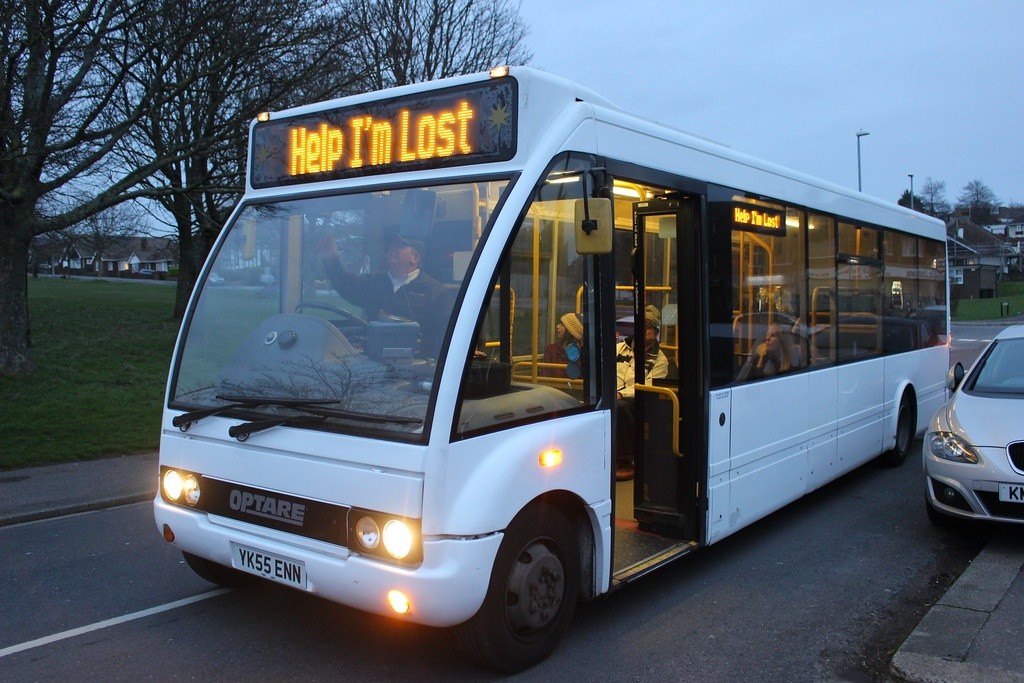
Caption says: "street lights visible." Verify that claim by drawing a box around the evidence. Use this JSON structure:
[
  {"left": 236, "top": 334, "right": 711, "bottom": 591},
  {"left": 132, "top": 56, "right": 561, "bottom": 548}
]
[
  {"left": 855, "top": 130, "right": 870, "bottom": 192},
  {"left": 908, "top": 174, "right": 916, "bottom": 211}
]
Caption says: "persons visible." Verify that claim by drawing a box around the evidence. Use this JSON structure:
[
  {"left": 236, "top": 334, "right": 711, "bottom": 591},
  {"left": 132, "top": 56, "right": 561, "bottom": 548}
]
[
  {"left": 755, "top": 283, "right": 796, "bottom": 318},
  {"left": 749, "top": 325, "right": 793, "bottom": 381},
  {"left": 609, "top": 305, "right": 670, "bottom": 479},
  {"left": 539, "top": 313, "right": 585, "bottom": 377},
  {"left": 322, "top": 230, "right": 445, "bottom": 361}
]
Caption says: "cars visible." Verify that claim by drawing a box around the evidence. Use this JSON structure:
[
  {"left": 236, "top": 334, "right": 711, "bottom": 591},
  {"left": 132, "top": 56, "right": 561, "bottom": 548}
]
[
  {"left": 131, "top": 269, "right": 154, "bottom": 279},
  {"left": 733, "top": 312, "right": 810, "bottom": 347},
  {"left": 921, "top": 324, "right": 1024, "bottom": 530},
  {"left": 206, "top": 272, "right": 225, "bottom": 286},
  {"left": 28, "top": 263, "right": 51, "bottom": 274}
]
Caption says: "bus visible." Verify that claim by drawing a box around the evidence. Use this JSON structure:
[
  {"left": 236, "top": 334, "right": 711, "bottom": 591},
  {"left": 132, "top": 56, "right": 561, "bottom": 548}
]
[{"left": 154, "top": 65, "right": 957, "bottom": 667}]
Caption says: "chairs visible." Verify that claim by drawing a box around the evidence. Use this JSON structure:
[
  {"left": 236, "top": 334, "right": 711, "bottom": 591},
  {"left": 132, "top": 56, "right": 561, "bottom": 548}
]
[{"left": 753, "top": 344, "right": 805, "bottom": 369}]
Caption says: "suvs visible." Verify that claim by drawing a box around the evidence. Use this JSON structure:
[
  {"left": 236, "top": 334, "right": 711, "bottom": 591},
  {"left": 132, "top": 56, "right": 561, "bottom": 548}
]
[{"left": 916, "top": 305, "right": 947, "bottom": 344}]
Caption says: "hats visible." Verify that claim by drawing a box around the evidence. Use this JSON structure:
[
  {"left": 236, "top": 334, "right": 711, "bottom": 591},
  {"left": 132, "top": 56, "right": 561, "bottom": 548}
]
[
  {"left": 392, "top": 232, "right": 425, "bottom": 257},
  {"left": 560, "top": 312, "right": 583, "bottom": 341},
  {"left": 645, "top": 305, "right": 660, "bottom": 330}
]
